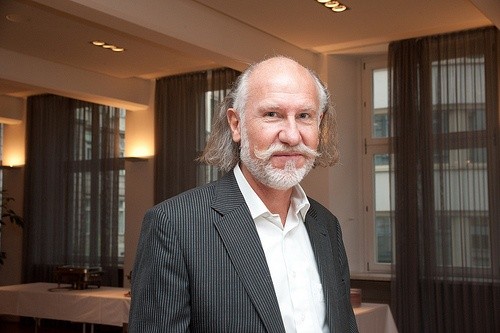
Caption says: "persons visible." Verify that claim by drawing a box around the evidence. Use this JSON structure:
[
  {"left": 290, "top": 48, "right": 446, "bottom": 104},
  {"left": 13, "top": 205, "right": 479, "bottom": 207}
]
[{"left": 127, "top": 54, "right": 361, "bottom": 333}]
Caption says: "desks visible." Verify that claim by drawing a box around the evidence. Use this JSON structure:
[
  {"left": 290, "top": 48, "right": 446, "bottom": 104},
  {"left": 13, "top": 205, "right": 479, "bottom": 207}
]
[{"left": 0, "top": 281, "right": 401, "bottom": 333}]
